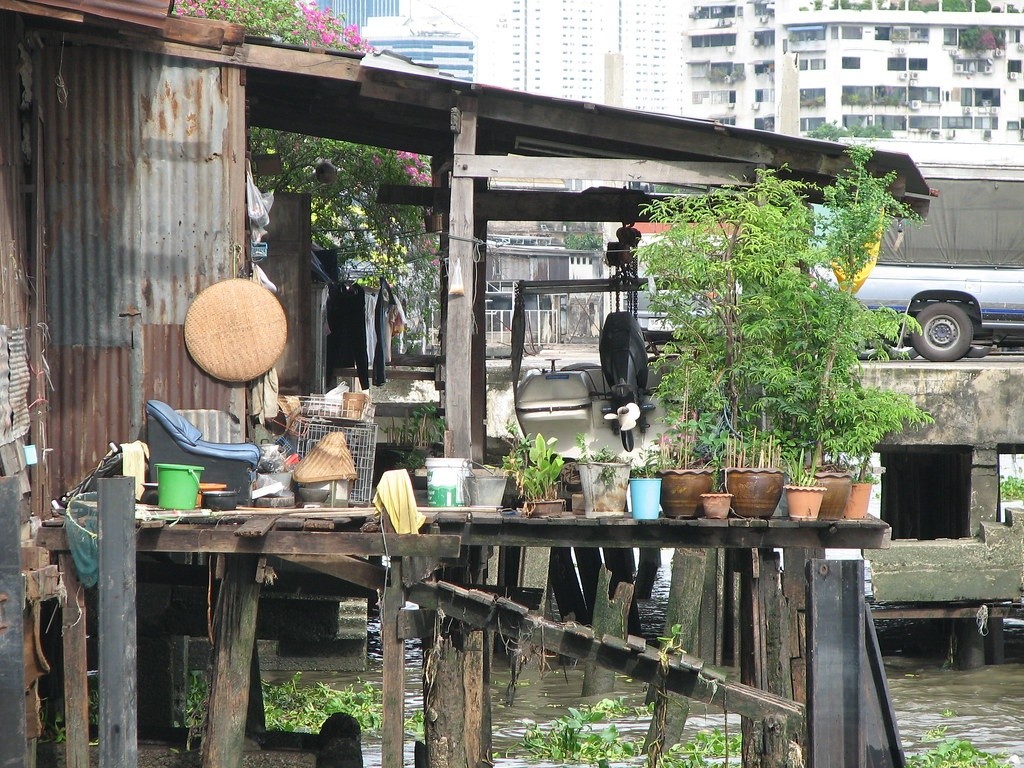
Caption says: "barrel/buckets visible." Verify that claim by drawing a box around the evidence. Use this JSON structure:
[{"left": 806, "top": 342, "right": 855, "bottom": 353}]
[
  {"left": 154, "top": 463, "right": 204, "bottom": 510},
  {"left": 425, "top": 456, "right": 466, "bottom": 506}
]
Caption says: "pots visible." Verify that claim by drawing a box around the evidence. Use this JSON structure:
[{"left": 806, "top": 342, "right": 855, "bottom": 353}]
[{"left": 201, "top": 488, "right": 238, "bottom": 510}]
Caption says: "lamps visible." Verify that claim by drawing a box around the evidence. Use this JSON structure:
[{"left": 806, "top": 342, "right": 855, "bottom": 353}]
[{"left": 272, "top": 162, "right": 336, "bottom": 194}]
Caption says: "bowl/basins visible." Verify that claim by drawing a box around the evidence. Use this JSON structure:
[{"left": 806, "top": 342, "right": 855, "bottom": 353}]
[{"left": 198, "top": 484, "right": 227, "bottom": 507}]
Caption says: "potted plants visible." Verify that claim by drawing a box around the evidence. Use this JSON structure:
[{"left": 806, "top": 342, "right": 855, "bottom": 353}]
[
  {"left": 783, "top": 448, "right": 828, "bottom": 522},
  {"left": 721, "top": 427, "right": 785, "bottom": 519},
  {"left": 628, "top": 451, "right": 664, "bottom": 519},
  {"left": 808, "top": 457, "right": 854, "bottom": 519},
  {"left": 499, "top": 418, "right": 634, "bottom": 518},
  {"left": 700, "top": 459, "right": 733, "bottom": 519},
  {"left": 424, "top": 212, "right": 443, "bottom": 232},
  {"left": 654, "top": 431, "right": 714, "bottom": 520},
  {"left": 811, "top": 387, "right": 935, "bottom": 520},
  {"left": 380, "top": 402, "right": 447, "bottom": 490}
]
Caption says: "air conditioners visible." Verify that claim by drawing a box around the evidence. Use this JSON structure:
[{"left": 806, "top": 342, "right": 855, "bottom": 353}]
[
  {"left": 993, "top": 48, "right": 1005, "bottom": 57},
  {"left": 953, "top": 64, "right": 963, "bottom": 73},
  {"left": 1009, "top": 71, "right": 1018, "bottom": 80},
  {"left": 728, "top": 46, "right": 735, "bottom": 52},
  {"left": 1018, "top": 42, "right": 1024, "bottom": 51},
  {"left": 713, "top": 8, "right": 722, "bottom": 13},
  {"left": 990, "top": 106, "right": 999, "bottom": 115},
  {"left": 964, "top": 106, "right": 972, "bottom": 114},
  {"left": 751, "top": 102, "right": 759, "bottom": 110},
  {"left": 764, "top": 68, "right": 770, "bottom": 74},
  {"left": 984, "top": 66, "right": 993, "bottom": 74},
  {"left": 723, "top": 75, "right": 732, "bottom": 84},
  {"left": 760, "top": 16, "right": 768, "bottom": 22},
  {"left": 897, "top": 47, "right": 906, "bottom": 53},
  {"left": 911, "top": 72, "right": 919, "bottom": 79},
  {"left": 899, "top": 72, "right": 908, "bottom": 80},
  {"left": 720, "top": 19, "right": 731, "bottom": 25},
  {"left": 728, "top": 103, "right": 733, "bottom": 107},
  {"left": 911, "top": 100, "right": 921, "bottom": 110},
  {"left": 752, "top": 39, "right": 759, "bottom": 46}
]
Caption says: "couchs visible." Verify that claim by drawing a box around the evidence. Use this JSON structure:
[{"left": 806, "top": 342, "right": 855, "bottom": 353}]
[{"left": 145, "top": 400, "right": 260, "bottom": 507}]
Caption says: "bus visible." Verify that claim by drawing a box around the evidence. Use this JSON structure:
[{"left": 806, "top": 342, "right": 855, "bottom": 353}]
[{"left": 807, "top": 132, "right": 1024, "bottom": 364}]
